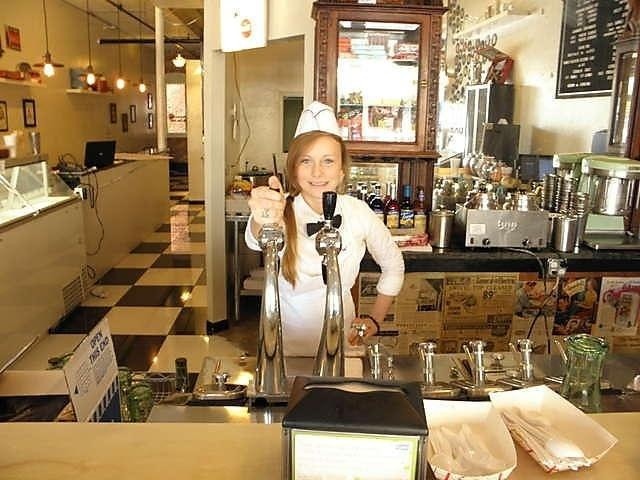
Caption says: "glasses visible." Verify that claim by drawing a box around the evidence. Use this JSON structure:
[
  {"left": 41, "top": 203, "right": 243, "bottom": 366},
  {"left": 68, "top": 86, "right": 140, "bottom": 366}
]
[{"left": 297, "top": 159, "right": 338, "bottom": 168}]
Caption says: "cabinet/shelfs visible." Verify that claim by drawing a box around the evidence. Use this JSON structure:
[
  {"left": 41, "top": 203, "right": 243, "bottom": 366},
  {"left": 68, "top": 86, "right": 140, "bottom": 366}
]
[
  {"left": 310, "top": 0, "right": 449, "bottom": 210},
  {"left": 464, "top": 82, "right": 514, "bottom": 160},
  {"left": 0, "top": 153, "right": 94, "bottom": 375}
]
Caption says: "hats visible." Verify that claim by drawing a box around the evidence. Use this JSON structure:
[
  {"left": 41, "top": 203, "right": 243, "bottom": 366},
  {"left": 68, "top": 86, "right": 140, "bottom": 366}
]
[{"left": 294, "top": 101, "right": 342, "bottom": 138}]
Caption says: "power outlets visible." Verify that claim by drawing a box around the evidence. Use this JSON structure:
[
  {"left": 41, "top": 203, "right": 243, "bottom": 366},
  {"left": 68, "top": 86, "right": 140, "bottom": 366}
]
[{"left": 546, "top": 258, "right": 568, "bottom": 280}]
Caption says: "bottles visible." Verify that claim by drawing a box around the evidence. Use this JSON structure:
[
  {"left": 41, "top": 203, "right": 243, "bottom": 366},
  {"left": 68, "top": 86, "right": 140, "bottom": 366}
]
[
  {"left": 430, "top": 205, "right": 456, "bottom": 248},
  {"left": 28, "top": 132, "right": 40, "bottom": 155},
  {"left": 176, "top": 356, "right": 189, "bottom": 395},
  {"left": 551, "top": 209, "right": 578, "bottom": 253},
  {"left": 463, "top": 152, "right": 513, "bottom": 184},
  {"left": 344, "top": 181, "right": 429, "bottom": 232}
]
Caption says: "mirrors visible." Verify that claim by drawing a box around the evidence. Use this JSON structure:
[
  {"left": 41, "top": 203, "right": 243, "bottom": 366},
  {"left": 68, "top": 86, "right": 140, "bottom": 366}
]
[
  {"left": 332, "top": 15, "right": 423, "bottom": 148},
  {"left": 343, "top": 160, "right": 401, "bottom": 208}
]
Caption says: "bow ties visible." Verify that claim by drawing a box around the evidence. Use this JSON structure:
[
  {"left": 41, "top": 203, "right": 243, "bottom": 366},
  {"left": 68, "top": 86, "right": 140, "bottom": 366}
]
[{"left": 307, "top": 214, "right": 342, "bottom": 237}]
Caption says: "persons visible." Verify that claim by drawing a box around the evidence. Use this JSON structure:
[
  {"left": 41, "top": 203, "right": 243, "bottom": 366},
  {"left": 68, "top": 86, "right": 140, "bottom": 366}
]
[
  {"left": 575, "top": 277, "right": 598, "bottom": 312},
  {"left": 515, "top": 280, "right": 541, "bottom": 315},
  {"left": 555, "top": 292, "right": 576, "bottom": 328},
  {"left": 245, "top": 130, "right": 407, "bottom": 359}
]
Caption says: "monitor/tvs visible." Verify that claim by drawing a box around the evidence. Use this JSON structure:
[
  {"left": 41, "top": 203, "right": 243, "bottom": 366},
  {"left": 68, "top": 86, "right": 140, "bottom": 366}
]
[{"left": 84, "top": 140, "right": 116, "bottom": 167}]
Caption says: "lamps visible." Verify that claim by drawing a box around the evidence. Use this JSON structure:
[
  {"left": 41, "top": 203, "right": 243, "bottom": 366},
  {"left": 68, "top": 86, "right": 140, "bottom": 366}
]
[
  {"left": 171, "top": 45, "right": 187, "bottom": 70},
  {"left": 112, "top": 1, "right": 127, "bottom": 90},
  {"left": 77, "top": 1, "right": 104, "bottom": 89},
  {"left": 135, "top": 0, "right": 149, "bottom": 94},
  {"left": 30, "top": 1, "right": 65, "bottom": 77}
]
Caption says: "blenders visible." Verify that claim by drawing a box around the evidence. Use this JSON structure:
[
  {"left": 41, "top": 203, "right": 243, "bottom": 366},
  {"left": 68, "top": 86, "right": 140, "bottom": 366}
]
[{"left": 552, "top": 151, "right": 594, "bottom": 187}]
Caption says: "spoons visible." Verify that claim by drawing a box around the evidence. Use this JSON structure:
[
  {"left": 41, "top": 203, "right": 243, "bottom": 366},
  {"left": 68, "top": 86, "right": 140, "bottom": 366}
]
[
  {"left": 500, "top": 407, "right": 590, "bottom": 467},
  {"left": 426, "top": 424, "right": 505, "bottom": 475}
]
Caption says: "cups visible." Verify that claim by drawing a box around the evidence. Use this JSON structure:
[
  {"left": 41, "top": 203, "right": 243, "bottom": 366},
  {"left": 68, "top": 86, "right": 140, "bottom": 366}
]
[
  {"left": 559, "top": 334, "right": 608, "bottom": 414},
  {"left": 125, "top": 383, "right": 155, "bottom": 421},
  {"left": 450, "top": 158, "right": 462, "bottom": 175},
  {"left": 117, "top": 367, "right": 132, "bottom": 421},
  {"left": 542, "top": 173, "right": 590, "bottom": 244}
]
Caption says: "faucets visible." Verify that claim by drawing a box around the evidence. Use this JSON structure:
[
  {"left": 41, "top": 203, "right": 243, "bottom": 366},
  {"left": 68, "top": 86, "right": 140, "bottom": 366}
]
[
  {"left": 257, "top": 186, "right": 288, "bottom": 397},
  {"left": 367, "top": 338, "right": 569, "bottom": 399},
  {"left": 312, "top": 190, "right": 346, "bottom": 379}
]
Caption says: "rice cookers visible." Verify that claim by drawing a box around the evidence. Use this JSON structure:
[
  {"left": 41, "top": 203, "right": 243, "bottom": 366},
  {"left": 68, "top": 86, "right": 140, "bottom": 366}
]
[{"left": 239, "top": 170, "right": 273, "bottom": 188}]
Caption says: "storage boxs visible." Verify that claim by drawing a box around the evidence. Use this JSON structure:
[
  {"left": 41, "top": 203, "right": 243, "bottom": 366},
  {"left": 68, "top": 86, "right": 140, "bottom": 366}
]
[{"left": 1, "top": 317, "right": 124, "bottom": 423}]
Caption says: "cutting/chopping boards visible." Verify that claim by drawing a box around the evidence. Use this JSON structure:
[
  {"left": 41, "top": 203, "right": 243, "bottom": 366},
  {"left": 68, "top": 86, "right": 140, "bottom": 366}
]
[{"left": 391, "top": 236, "right": 434, "bottom": 253}]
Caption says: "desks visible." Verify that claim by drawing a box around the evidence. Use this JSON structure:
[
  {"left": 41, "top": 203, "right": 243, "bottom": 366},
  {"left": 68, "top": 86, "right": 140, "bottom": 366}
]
[{"left": 225, "top": 213, "right": 264, "bottom": 323}]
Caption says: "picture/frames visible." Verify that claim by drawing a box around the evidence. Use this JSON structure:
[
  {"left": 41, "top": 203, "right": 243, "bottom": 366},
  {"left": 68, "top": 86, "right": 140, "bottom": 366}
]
[
  {"left": 110, "top": 91, "right": 154, "bottom": 134},
  {"left": 0, "top": 101, "right": 9, "bottom": 132},
  {"left": 22, "top": 98, "right": 38, "bottom": 127}
]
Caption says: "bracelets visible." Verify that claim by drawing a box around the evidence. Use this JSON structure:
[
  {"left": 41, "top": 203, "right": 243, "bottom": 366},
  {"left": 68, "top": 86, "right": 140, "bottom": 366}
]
[{"left": 369, "top": 316, "right": 381, "bottom": 333}]
